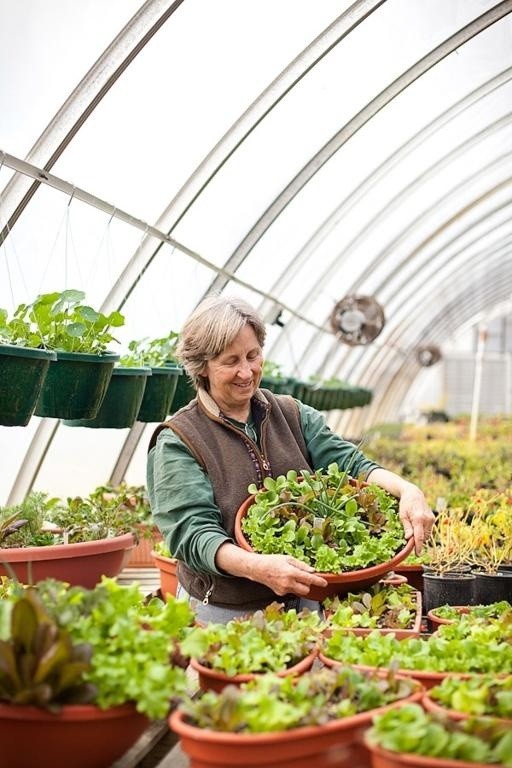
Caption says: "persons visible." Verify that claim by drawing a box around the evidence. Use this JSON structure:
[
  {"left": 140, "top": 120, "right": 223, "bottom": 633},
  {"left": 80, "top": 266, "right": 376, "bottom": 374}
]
[{"left": 146, "top": 294, "right": 436, "bottom": 627}]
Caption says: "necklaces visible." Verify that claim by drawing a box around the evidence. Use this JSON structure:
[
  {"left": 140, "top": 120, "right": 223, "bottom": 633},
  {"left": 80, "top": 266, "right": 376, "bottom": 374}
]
[{"left": 243, "top": 439, "right": 272, "bottom": 489}]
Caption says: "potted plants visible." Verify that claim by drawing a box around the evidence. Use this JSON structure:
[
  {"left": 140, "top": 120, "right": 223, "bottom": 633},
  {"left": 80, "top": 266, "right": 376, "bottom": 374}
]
[
  {"left": 262, "top": 359, "right": 376, "bottom": 412},
  {"left": 0, "top": 286, "right": 192, "bottom": 432},
  {"left": 1, "top": 459, "right": 510, "bottom": 767}
]
